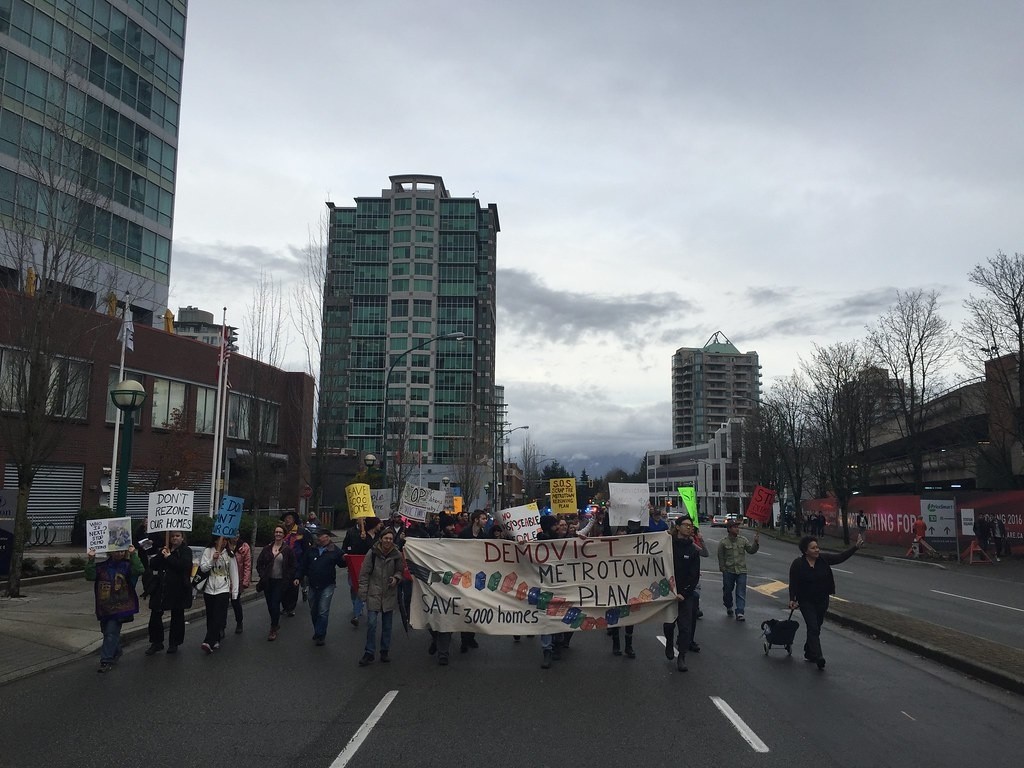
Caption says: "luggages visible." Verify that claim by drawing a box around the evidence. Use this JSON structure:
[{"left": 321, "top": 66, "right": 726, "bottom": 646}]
[{"left": 760, "top": 604, "right": 800, "bottom": 655}]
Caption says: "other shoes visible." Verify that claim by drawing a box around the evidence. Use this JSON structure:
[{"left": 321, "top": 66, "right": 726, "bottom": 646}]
[
  {"left": 312, "top": 634, "right": 325, "bottom": 646},
  {"left": 200, "top": 640, "right": 220, "bottom": 654},
  {"left": 219, "top": 625, "right": 226, "bottom": 638},
  {"left": 280, "top": 607, "right": 296, "bottom": 616},
  {"left": 697, "top": 608, "right": 703, "bottom": 620},
  {"left": 97, "top": 646, "right": 123, "bottom": 673},
  {"left": 235, "top": 621, "right": 244, "bottom": 634},
  {"left": 803, "top": 649, "right": 826, "bottom": 670},
  {"left": 351, "top": 617, "right": 361, "bottom": 626},
  {"left": 689, "top": 641, "right": 701, "bottom": 653},
  {"left": 145, "top": 643, "right": 178, "bottom": 655},
  {"left": 666, "top": 637, "right": 688, "bottom": 672},
  {"left": 428, "top": 632, "right": 573, "bottom": 669},
  {"left": 358, "top": 649, "right": 392, "bottom": 664},
  {"left": 267, "top": 625, "right": 280, "bottom": 641},
  {"left": 726, "top": 606, "right": 745, "bottom": 621}
]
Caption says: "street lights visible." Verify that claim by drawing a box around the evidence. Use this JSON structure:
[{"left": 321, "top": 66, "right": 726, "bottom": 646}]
[
  {"left": 494, "top": 425, "right": 529, "bottom": 512},
  {"left": 381, "top": 332, "right": 465, "bottom": 502},
  {"left": 520, "top": 489, "right": 526, "bottom": 505},
  {"left": 688, "top": 458, "right": 721, "bottom": 514},
  {"left": 109, "top": 379, "right": 149, "bottom": 518},
  {"left": 363, "top": 454, "right": 377, "bottom": 476}
]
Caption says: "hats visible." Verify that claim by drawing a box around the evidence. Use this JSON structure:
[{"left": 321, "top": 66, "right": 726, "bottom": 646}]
[
  {"left": 726, "top": 518, "right": 741, "bottom": 528},
  {"left": 280, "top": 511, "right": 300, "bottom": 522},
  {"left": 654, "top": 507, "right": 661, "bottom": 517},
  {"left": 540, "top": 515, "right": 558, "bottom": 532},
  {"left": 305, "top": 529, "right": 339, "bottom": 537}
]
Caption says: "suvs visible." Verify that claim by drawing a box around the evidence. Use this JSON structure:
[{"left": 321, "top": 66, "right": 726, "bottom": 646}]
[{"left": 724, "top": 513, "right": 747, "bottom": 523}]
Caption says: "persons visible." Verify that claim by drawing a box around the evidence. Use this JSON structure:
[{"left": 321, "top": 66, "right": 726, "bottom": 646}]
[
  {"left": 802, "top": 510, "right": 826, "bottom": 539},
  {"left": 912, "top": 515, "right": 927, "bottom": 537},
  {"left": 973, "top": 512, "right": 1006, "bottom": 562},
  {"left": 341, "top": 507, "right": 709, "bottom": 671},
  {"left": 856, "top": 510, "right": 868, "bottom": 535},
  {"left": 718, "top": 520, "right": 761, "bottom": 621},
  {"left": 83, "top": 511, "right": 348, "bottom": 673},
  {"left": 788, "top": 536, "right": 864, "bottom": 673}
]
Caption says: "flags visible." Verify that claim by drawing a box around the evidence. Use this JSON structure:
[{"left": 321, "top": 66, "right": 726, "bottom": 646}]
[
  {"left": 215, "top": 326, "right": 232, "bottom": 389},
  {"left": 116, "top": 310, "right": 135, "bottom": 351}
]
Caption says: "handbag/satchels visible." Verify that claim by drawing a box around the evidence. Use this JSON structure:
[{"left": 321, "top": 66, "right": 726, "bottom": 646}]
[{"left": 192, "top": 566, "right": 211, "bottom": 591}]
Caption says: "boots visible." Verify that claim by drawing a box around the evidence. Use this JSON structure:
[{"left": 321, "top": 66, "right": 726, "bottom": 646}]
[{"left": 612, "top": 633, "right": 636, "bottom": 658}]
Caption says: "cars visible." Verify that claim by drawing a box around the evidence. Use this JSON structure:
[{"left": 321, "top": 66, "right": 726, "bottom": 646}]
[{"left": 710, "top": 515, "right": 728, "bottom": 528}]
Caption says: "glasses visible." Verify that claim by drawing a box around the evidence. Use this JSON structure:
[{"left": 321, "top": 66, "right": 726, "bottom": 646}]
[{"left": 679, "top": 523, "right": 693, "bottom": 528}]
